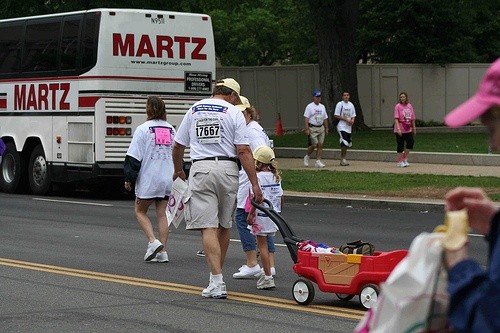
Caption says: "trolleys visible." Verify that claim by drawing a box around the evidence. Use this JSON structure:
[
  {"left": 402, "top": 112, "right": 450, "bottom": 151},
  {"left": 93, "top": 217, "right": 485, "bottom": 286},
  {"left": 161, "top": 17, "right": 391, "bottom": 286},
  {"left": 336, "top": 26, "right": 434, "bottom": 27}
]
[{"left": 249, "top": 197, "right": 410, "bottom": 310}]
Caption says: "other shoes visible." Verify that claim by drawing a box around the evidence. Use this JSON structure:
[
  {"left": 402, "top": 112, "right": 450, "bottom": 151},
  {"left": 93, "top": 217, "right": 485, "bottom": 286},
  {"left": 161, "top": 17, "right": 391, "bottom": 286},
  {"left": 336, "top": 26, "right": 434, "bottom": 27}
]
[
  {"left": 398, "top": 160, "right": 410, "bottom": 167},
  {"left": 257, "top": 275, "right": 276, "bottom": 290},
  {"left": 340, "top": 161, "right": 350, "bottom": 166},
  {"left": 202, "top": 277, "right": 228, "bottom": 298},
  {"left": 262, "top": 267, "right": 276, "bottom": 275},
  {"left": 303, "top": 155, "right": 310, "bottom": 167},
  {"left": 314, "top": 162, "right": 325, "bottom": 168},
  {"left": 233, "top": 264, "right": 260, "bottom": 279},
  {"left": 196, "top": 250, "right": 205, "bottom": 257}
]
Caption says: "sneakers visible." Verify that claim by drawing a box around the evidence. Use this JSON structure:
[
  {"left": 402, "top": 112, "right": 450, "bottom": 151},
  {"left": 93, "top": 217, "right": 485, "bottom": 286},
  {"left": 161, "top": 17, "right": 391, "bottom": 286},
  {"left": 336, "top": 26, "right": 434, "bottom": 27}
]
[
  {"left": 144, "top": 239, "right": 164, "bottom": 261},
  {"left": 151, "top": 251, "right": 169, "bottom": 263}
]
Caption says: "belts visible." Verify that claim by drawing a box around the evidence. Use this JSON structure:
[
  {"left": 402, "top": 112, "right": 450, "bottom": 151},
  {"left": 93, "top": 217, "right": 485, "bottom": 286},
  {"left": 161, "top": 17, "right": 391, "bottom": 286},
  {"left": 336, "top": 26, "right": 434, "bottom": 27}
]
[
  {"left": 312, "top": 123, "right": 322, "bottom": 127},
  {"left": 193, "top": 156, "right": 240, "bottom": 163}
]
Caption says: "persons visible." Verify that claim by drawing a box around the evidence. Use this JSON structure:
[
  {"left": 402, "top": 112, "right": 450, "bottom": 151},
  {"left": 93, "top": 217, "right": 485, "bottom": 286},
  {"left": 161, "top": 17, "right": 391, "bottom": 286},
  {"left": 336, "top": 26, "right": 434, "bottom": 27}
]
[
  {"left": 334, "top": 91, "right": 356, "bottom": 166},
  {"left": 123, "top": 96, "right": 177, "bottom": 263},
  {"left": 246, "top": 145, "right": 284, "bottom": 289},
  {"left": 303, "top": 89, "right": 329, "bottom": 168},
  {"left": 232, "top": 95, "right": 276, "bottom": 278},
  {"left": 393, "top": 93, "right": 416, "bottom": 168},
  {"left": 172, "top": 78, "right": 264, "bottom": 298},
  {"left": 445, "top": 59, "right": 500, "bottom": 333}
]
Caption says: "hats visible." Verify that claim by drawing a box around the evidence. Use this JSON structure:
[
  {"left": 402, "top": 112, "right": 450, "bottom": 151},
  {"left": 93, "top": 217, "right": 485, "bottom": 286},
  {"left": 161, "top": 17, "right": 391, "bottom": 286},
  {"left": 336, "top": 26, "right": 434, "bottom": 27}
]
[
  {"left": 314, "top": 90, "right": 322, "bottom": 96},
  {"left": 253, "top": 145, "right": 275, "bottom": 163},
  {"left": 234, "top": 96, "right": 252, "bottom": 111},
  {"left": 443, "top": 57, "right": 500, "bottom": 127},
  {"left": 211, "top": 77, "right": 243, "bottom": 106}
]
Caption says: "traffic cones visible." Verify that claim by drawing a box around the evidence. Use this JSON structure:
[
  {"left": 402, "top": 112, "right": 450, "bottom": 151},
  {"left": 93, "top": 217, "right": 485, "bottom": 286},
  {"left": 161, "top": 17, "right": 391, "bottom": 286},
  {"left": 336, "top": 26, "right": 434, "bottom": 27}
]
[{"left": 274, "top": 112, "right": 285, "bottom": 136}]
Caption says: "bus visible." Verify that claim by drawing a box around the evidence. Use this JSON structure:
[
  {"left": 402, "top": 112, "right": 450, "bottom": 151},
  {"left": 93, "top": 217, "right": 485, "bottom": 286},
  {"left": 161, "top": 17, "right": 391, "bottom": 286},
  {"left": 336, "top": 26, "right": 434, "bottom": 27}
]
[{"left": 0, "top": 8, "right": 216, "bottom": 196}]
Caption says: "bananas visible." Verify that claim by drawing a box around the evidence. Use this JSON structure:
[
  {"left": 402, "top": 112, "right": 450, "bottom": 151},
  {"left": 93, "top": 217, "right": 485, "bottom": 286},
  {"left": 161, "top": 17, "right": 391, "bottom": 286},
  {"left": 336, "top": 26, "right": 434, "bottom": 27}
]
[{"left": 434, "top": 209, "right": 469, "bottom": 251}]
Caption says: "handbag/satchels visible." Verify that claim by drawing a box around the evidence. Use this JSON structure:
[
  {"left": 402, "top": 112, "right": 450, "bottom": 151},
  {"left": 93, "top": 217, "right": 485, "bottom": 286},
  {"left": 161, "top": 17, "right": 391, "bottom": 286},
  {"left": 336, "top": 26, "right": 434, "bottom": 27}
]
[
  {"left": 403, "top": 121, "right": 412, "bottom": 130},
  {"left": 333, "top": 118, "right": 340, "bottom": 125},
  {"left": 353, "top": 231, "right": 444, "bottom": 333}
]
